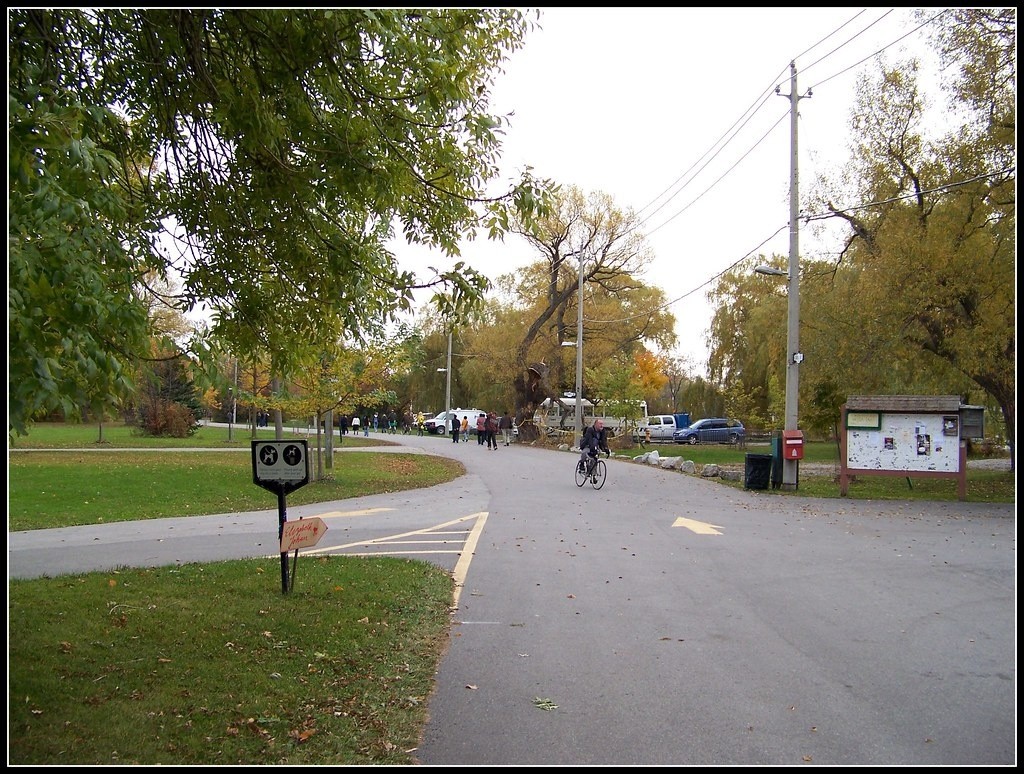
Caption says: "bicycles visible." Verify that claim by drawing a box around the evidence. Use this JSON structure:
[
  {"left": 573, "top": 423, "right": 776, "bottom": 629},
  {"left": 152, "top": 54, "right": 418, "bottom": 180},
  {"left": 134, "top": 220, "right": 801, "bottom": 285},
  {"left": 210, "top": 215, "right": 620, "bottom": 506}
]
[
  {"left": 416, "top": 424, "right": 424, "bottom": 436},
  {"left": 401, "top": 421, "right": 411, "bottom": 435},
  {"left": 574, "top": 448, "right": 608, "bottom": 490},
  {"left": 388, "top": 420, "right": 397, "bottom": 434}
]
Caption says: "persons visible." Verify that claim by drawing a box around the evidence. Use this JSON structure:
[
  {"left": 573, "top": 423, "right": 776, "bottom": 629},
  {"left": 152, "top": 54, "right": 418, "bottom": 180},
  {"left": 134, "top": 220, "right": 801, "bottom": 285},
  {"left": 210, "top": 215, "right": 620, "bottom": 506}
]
[
  {"left": 452, "top": 414, "right": 460, "bottom": 443},
  {"left": 477, "top": 411, "right": 513, "bottom": 451},
  {"left": 578, "top": 419, "right": 609, "bottom": 484},
  {"left": 461, "top": 416, "right": 469, "bottom": 442},
  {"left": 341, "top": 409, "right": 424, "bottom": 438},
  {"left": 257, "top": 411, "right": 270, "bottom": 427}
]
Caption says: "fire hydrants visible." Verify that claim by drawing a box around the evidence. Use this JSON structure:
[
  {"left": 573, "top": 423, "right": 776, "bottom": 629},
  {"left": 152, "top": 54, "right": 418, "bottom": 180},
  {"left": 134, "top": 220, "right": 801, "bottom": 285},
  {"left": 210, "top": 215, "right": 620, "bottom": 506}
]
[{"left": 644, "top": 428, "right": 653, "bottom": 443}]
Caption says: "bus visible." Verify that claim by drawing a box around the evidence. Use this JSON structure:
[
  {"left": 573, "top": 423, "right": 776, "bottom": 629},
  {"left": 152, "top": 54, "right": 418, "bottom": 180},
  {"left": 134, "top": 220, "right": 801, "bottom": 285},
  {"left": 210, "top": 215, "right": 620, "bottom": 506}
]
[{"left": 532, "top": 392, "right": 647, "bottom": 439}]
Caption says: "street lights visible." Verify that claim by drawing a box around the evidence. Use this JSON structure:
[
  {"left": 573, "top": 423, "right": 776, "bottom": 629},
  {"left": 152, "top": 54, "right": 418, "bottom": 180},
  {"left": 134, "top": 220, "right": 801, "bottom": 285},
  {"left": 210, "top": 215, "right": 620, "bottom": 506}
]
[
  {"left": 228, "top": 388, "right": 237, "bottom": 424},
  {"left": 755, "top": 264, "right": 800, "bottom": 493},
  {"left": 560, "top": 340, "right": 583, "bottom": 448},
  {"left": 437, "top": 368, "right": 451, "bottom": 439}
]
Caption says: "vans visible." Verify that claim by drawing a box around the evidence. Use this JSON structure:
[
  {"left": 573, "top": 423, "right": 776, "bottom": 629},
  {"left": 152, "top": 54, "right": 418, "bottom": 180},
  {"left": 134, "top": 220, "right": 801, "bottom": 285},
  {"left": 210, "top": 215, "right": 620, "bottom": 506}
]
[
  {"left": 425, "top": 407, "right": 486, "bottom": 436},
  {"left": 635, "top": 414, "right": 677, "bottom": 443}
]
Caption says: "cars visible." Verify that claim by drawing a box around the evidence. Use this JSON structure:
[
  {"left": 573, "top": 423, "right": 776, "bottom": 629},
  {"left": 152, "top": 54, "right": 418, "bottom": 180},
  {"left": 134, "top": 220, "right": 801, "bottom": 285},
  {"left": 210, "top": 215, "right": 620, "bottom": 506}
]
[
  {"left": 672, "top": 417, "right": 746, "bottom": 445},
  {"left": 494, "top": 417, "right": 519, "bottom": 436}
]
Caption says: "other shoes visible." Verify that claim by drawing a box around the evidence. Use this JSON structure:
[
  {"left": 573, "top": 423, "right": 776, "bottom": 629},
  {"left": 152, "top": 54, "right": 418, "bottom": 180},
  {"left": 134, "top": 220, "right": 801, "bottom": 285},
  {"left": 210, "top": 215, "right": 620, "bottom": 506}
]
[
  {"left": 494, "top": 446, "right": 498, "bottom": 450},
  {"left": 463, "top": 439, "right": 468, "bottom": 443},
  {"left": 590, "top": 477, "right": 598, "bottom": 484},
  {"left": 579, "top": 463, "right": 585, "bottom": 473},
  {"left": 488, "top": 446, "right": 491, "bottom": 450},
  {"left": 504, "top": 443, "right": 510, "bottom": 446}
]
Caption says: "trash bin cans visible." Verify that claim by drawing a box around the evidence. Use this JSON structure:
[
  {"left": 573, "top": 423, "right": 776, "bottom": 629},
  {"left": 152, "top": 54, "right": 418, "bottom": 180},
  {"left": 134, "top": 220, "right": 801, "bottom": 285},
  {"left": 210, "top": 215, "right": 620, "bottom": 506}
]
[{"left": 743, "top": 453, "right": 773, "bottom": 492}]
